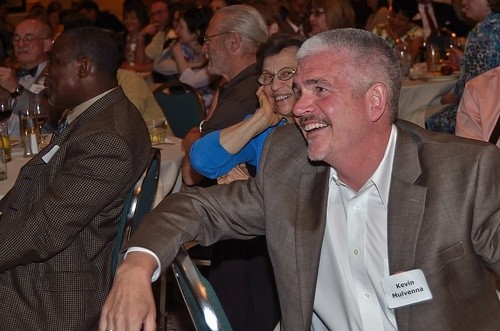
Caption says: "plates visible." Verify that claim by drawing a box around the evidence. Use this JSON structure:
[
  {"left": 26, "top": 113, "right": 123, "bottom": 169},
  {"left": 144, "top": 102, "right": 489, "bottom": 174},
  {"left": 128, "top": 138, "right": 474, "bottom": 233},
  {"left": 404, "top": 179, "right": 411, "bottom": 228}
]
[{"left": 423, "top": 74, "right": 462, "bottom": 81}]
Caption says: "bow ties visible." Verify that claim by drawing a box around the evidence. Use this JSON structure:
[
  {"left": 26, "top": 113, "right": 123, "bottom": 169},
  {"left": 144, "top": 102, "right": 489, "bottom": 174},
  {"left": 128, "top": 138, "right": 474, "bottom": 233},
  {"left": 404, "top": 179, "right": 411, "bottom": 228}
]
[{"left": 15, "top": 66, "right": 38, "bottom": 77}]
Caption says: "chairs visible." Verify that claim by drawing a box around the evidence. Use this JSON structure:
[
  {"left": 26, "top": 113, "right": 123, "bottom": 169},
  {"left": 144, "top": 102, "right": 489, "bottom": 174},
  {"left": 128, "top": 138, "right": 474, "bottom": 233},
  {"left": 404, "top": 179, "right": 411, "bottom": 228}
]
[
  {"left": 455, "top": 66, "right": 500, "bottom": 147},
  {"left": 107, "top": 147, "right": 233, "bottom": 331}
]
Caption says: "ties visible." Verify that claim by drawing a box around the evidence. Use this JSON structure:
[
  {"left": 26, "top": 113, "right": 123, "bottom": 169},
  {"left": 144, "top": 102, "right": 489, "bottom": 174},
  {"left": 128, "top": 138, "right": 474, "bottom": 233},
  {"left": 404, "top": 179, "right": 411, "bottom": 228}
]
[{"left": 424, "top": 5, "right": 436, "bottom": 31}]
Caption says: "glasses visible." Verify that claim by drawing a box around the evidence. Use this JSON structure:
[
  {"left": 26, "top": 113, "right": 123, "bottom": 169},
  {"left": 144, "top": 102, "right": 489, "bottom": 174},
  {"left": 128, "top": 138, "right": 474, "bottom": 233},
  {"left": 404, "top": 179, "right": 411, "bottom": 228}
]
[
  {"left": 11, "top": 36, "right": 46, "bottom": 45},
  {"left": 257, "top": 67, "right": 295, "bottom": 86},
  {"left": 204, "top": 32, "right": 228, "bottom": 45},
  {"left": 307, "top": 10, "right": 326, "bottom": 18}
]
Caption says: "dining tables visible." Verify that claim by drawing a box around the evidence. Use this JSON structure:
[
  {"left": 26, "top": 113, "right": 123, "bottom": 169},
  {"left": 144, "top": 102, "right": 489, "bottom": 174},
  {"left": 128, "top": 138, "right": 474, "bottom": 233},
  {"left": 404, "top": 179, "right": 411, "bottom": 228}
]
[{"left": 396, "top": 70, "right": 462, "bottom": 131}]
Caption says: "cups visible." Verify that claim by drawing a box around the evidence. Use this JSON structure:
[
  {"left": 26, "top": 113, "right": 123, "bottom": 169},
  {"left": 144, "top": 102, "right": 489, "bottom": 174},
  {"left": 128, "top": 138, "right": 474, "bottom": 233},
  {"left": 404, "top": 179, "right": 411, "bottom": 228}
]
[
  {"left": 0, "top": 122, "right": 11, "bottom": 163},
  {"left": 0, "top": 149, "right": 7, "bottom": 181},
  {"left": 427, "top": 44, "right": 440, "bottom": 72},
  {"left": 18, "top": 110, "right": 42, "bottom": 147}
]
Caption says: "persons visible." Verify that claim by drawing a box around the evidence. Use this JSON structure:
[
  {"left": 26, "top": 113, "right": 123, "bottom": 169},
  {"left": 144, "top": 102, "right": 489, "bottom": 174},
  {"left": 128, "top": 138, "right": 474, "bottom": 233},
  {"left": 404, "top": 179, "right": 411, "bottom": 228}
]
[
  {"left": 98, "top": 28, "right": 500, "bottom": 331},
  {"left": 0, "top": 0, "right": 500, "bottom": 331}
]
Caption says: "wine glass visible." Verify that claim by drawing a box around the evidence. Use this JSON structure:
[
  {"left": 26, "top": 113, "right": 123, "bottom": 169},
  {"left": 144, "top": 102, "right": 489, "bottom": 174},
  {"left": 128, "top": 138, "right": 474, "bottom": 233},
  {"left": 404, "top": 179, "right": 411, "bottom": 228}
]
[
  {"left": 0, "top": 97, "right": 13, "bottom": 146},
  {"left": 29, "top": 95, "right": 49, "bottom": 144}
]
